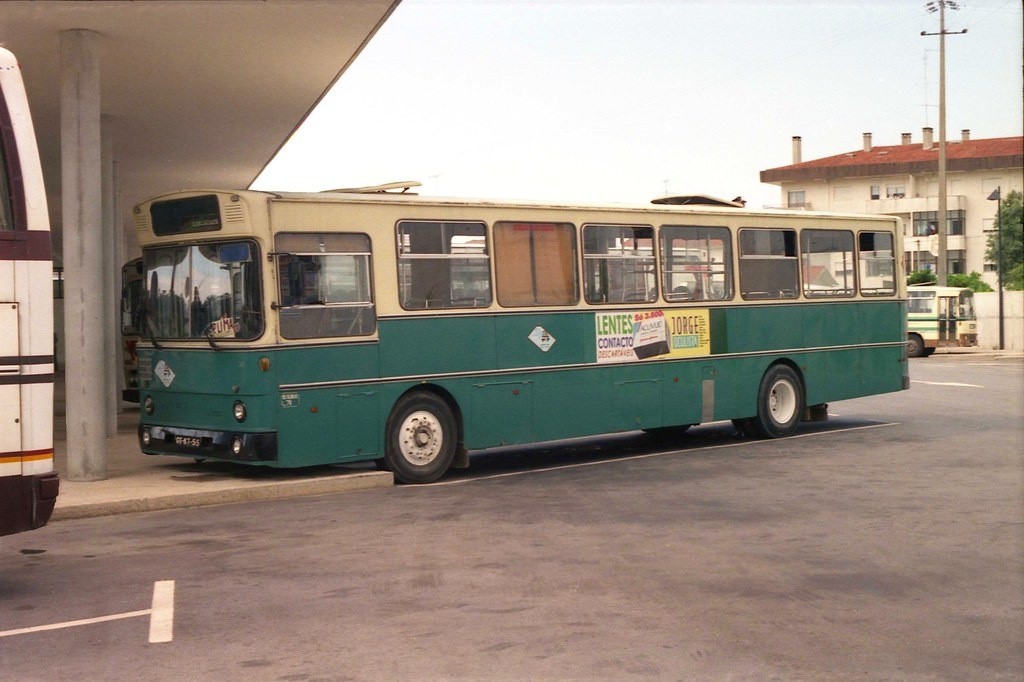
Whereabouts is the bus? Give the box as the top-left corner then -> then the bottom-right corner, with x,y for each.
741,283 -> 978,358
1,44 -> 64,537
119,179 -> 910,485
